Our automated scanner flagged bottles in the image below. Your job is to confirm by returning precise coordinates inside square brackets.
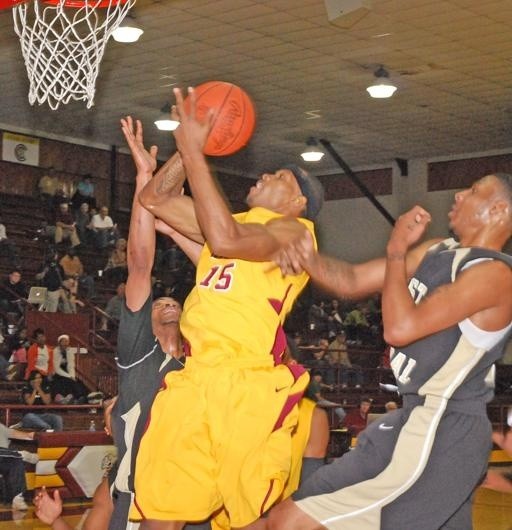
[89, 421, 96, 431]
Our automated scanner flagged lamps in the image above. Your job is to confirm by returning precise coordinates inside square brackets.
[108, 10, 145, 44]
[300, 137, 324, 162]
[366, 64, 397, 99]
[152, 102, 181, 131]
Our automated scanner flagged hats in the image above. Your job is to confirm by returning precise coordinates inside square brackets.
[281, 164, 325, 219]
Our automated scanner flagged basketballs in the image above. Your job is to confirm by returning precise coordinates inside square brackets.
[182, 83, 254, 156]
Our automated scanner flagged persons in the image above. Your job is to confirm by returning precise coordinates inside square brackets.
[1, 159, 511, 528]
[111, 115, 189, 528]
[129, 81, 326, 529]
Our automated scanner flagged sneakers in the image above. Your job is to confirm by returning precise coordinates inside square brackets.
[22, 451, 39, 464]
[12, 496, 28, 510]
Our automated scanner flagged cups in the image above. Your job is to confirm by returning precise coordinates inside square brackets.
[99, 270, 103, 276]
